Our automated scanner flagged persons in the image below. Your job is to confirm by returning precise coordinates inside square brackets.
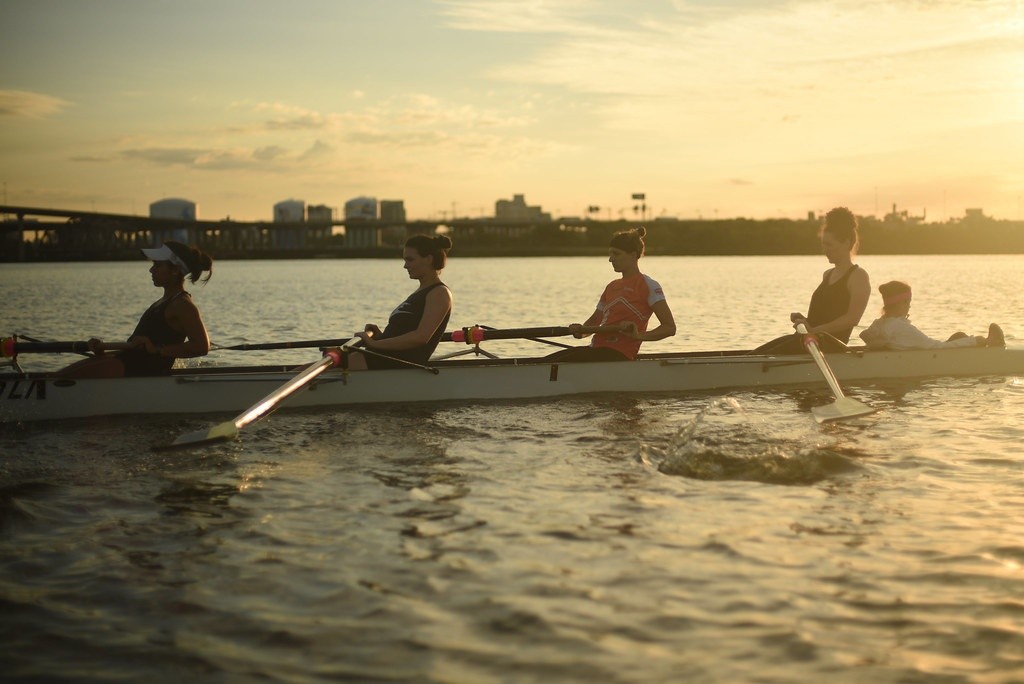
[289, 235, 453, 371]
[860, 281, 1005, 349]
[52, 241, 212, 377]
[538, 227, 676, 362]
[748, 208, 871, 355]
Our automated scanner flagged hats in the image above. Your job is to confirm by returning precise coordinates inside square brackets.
[140, 244, 191, 276]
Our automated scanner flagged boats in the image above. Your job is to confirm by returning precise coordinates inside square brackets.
[0, 345, 1024, 434]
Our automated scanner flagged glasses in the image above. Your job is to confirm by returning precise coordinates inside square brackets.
[152, 263, 160, 269]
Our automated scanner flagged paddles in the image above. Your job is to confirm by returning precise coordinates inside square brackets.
[794, 316, 876, 418]
[208, 322, 635, 353]
[148, 330, 375, 453]
[0, 337, 146, 357]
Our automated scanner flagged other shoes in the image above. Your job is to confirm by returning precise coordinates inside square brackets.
[986, 323, 1005, 346]
